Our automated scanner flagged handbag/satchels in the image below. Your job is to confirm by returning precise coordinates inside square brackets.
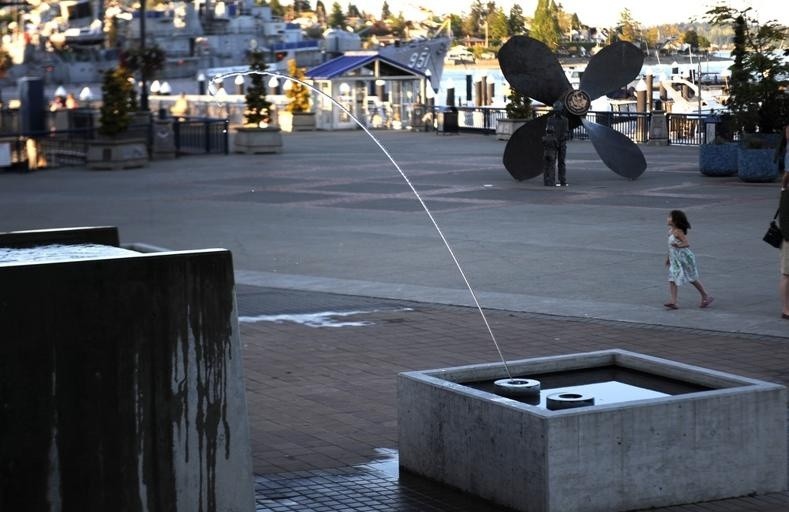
[763, 220, 782, 248]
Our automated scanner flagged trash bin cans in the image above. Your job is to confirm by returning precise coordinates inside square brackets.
[436, 105, 459, 137]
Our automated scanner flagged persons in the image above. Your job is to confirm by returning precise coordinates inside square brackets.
[546, 100, 569, 187]
[663, 209, 715, 310]
[542, 124, 560, 187]
[778, 170, 789, 319]
